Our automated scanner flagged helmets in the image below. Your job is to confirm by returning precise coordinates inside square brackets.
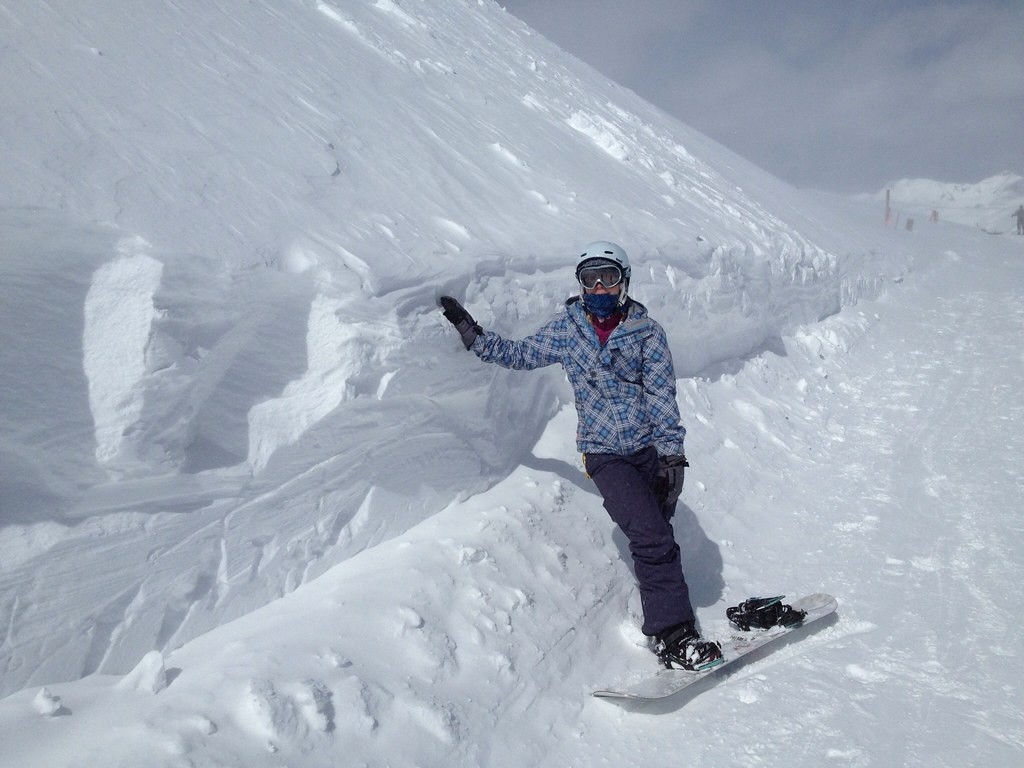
[575, 240, 631, 279]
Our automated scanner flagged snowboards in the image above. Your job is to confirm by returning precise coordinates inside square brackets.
[591, 589, 841, 705]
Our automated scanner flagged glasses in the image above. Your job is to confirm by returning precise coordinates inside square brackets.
[577, 265, 622, 289]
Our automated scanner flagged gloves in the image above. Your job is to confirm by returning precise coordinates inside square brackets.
[441, 295, 484, 351]
[653, 453, 690, 505]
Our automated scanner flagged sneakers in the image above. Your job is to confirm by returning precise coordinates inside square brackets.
[655, 622, 724, 671]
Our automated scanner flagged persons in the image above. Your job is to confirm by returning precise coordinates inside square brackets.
[1011, 205, 1024, 235]
[440, 239, 724, 671]
